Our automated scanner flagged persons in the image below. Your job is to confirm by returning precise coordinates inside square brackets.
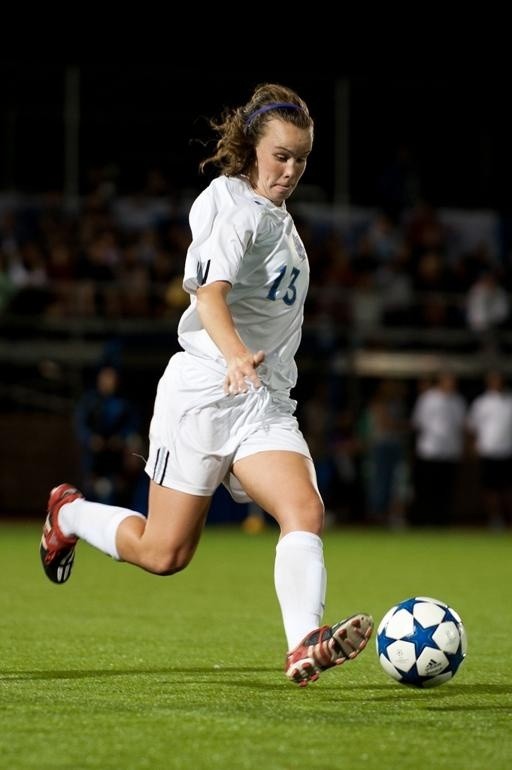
[37, 82, 374, 694]
[0, 174, 512, 530]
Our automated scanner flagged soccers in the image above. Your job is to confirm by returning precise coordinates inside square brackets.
[376, 596, 469, 689]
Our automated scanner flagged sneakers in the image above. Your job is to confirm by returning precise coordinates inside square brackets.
[283, 612, 375, 687]
[38, 483, 86, 584]
